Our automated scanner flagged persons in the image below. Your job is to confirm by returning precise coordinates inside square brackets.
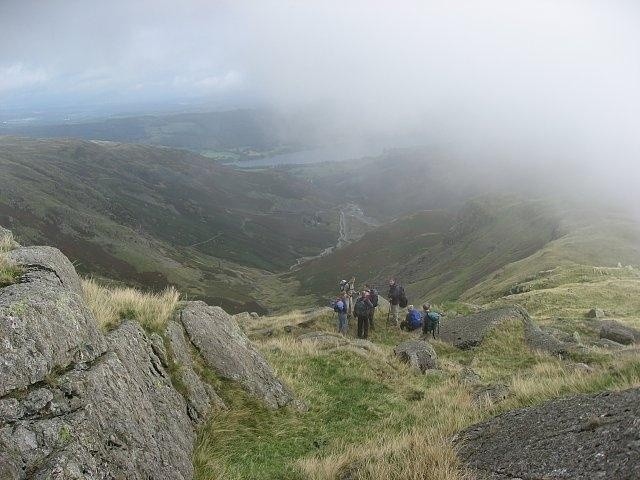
[335, 290, 353, 335]
[389, 278, 401, 327]
[342, 276, 360, 318]
[364, 284, 376, 331]
[418, 302, 433, 343]
[399, 305, 422, 332]
[353, 291, 374, 339]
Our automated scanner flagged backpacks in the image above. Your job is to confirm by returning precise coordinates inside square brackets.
[422, 312, 439, 333]
[353, 301, 370, 319]
[409, 310, 421, 328]
[371, 289, 378, 308]
[333, 296, 345, 312]
